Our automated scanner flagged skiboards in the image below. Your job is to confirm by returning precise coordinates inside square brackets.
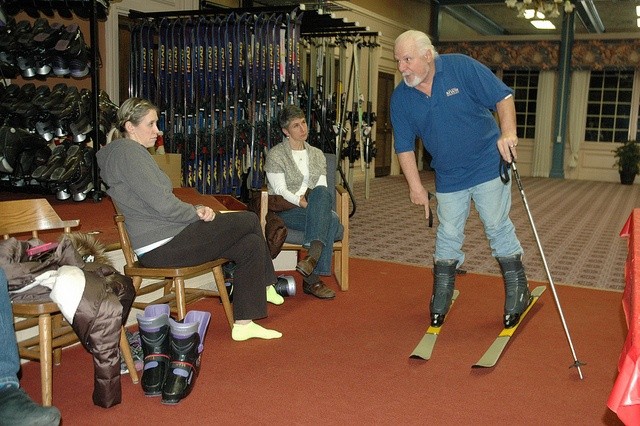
[409, 285, 547, 368]
[129, 12, 377, 203]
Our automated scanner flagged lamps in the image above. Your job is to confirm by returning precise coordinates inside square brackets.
[505, 0, 575, 21]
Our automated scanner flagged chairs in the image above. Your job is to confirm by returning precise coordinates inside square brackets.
[113, 214, 234, 332]
[257, 184, 350, 291]
[0, 198, 139, 407]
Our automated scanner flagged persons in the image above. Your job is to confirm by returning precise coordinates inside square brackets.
[96, 97, 285, 342]
[389, 29, 531, 316]
[0, 268, 62, 426]
[263, 104, 336, 299]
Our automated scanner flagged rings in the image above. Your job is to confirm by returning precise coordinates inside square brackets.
[514, 143, 517, 147]
[510, 145, 513, 148]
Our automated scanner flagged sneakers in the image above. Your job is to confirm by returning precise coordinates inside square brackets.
[0, 386, 61, 426]
[119, 332, 144, 375]
[0, 0, 117, 202]
[274, 275, 296, 297]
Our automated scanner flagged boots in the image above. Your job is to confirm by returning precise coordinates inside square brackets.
[495, 255, 531, 315]
[136, 304, 170, 396]
[162, 310, 211, 404]
[430, 259, 457, 316]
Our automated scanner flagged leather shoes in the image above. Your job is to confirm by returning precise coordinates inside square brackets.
[296, 255, 317, 277]
[303, 280, 336, 299]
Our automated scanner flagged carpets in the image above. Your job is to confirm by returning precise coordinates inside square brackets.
[1, 191, 627, 426]
